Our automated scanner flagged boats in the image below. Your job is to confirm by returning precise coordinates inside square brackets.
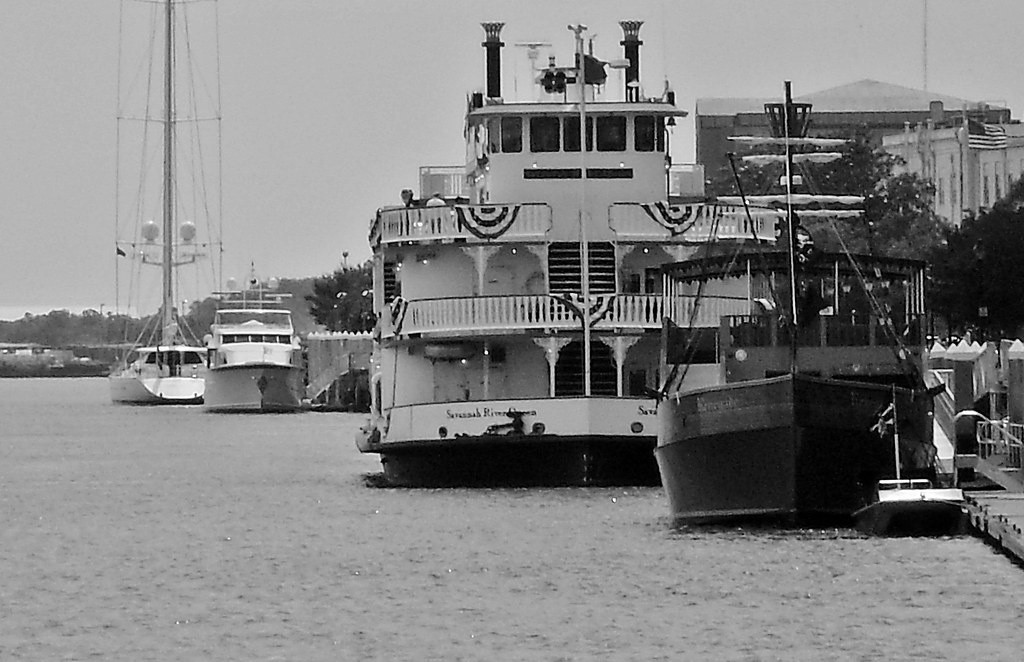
[49, 356, 111, 378]
[653, 101, 936, 533]
[354, 20, 777, 488]
[204, 261, 309, 413]
[850, 433, 964, 536]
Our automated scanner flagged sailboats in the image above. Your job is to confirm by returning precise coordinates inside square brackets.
[108, 1, 211, 403]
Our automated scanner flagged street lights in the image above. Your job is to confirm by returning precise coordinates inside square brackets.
[978, 303, 988, 344]
